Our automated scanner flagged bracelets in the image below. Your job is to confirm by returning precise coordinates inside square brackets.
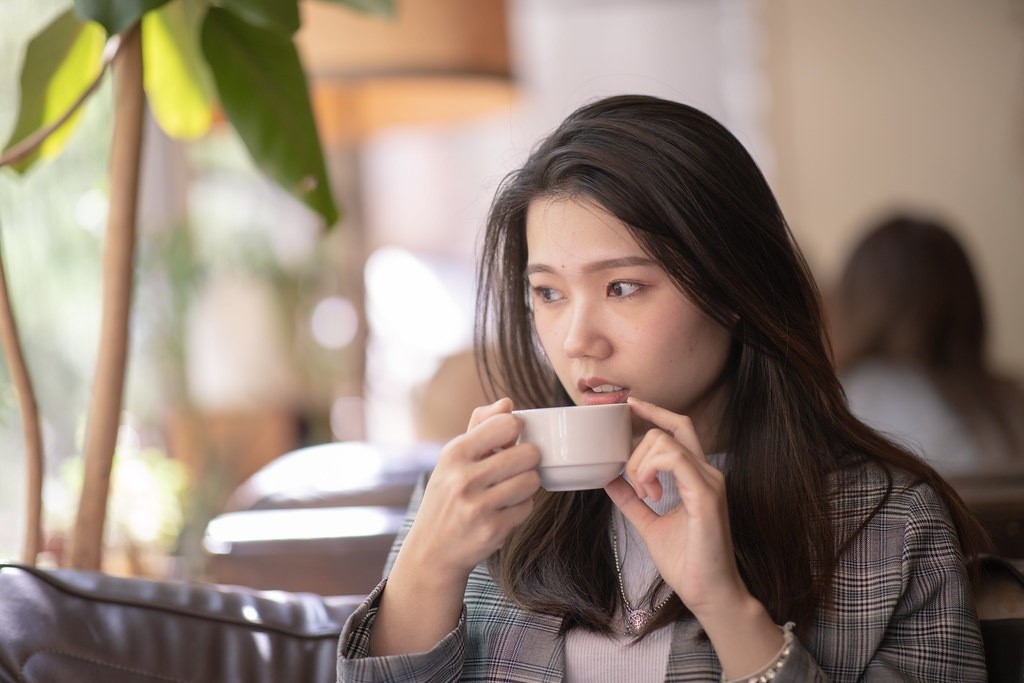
[741, 634, 790, 683]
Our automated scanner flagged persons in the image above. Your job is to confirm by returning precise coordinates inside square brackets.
[817, 205, 1024, 486]
[334, 93, 989, 683]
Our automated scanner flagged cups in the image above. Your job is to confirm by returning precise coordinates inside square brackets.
[503, 402, 634, 492]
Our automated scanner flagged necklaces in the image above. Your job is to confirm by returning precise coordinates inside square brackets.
[608, 502, 678, 636]
